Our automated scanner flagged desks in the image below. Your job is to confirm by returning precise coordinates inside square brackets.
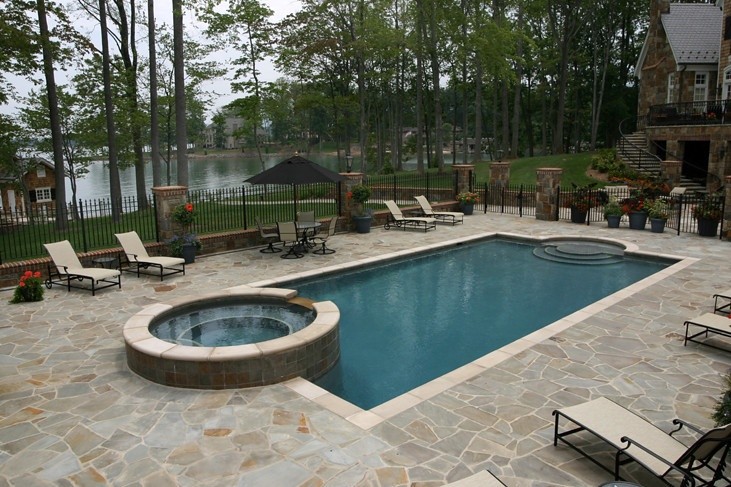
[276, 221, 321, 253]
[93, 257, 118, 280]
[598, 481, 644, 487]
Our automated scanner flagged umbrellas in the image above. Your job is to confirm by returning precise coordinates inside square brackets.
[243, 151, 347, 225]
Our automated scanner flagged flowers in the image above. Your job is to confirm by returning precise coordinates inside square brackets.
[346, 184, 372, 218]
[8, 271, 45, 304]
[571, 187, 721, 221]
[455, 190, 480, 205]
[170, 202, 203, 257]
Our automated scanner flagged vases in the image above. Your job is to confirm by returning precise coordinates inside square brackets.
[353, 217, 372, 233]
[461, 201, 474, 215]
[22, 287, 42, 300]
[571, 206, 718, 236]
[179, 244, 197, 264]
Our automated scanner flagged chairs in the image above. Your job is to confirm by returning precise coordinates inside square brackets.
[384, 195, 465, 233]
[552, 396, 731, 487]
[114, 230, 186, 281]
[440, 469, 508, 487]
[684, 288, 731, 353]
[255, 210, 338, 259]
[44, 239, 122, 296]
